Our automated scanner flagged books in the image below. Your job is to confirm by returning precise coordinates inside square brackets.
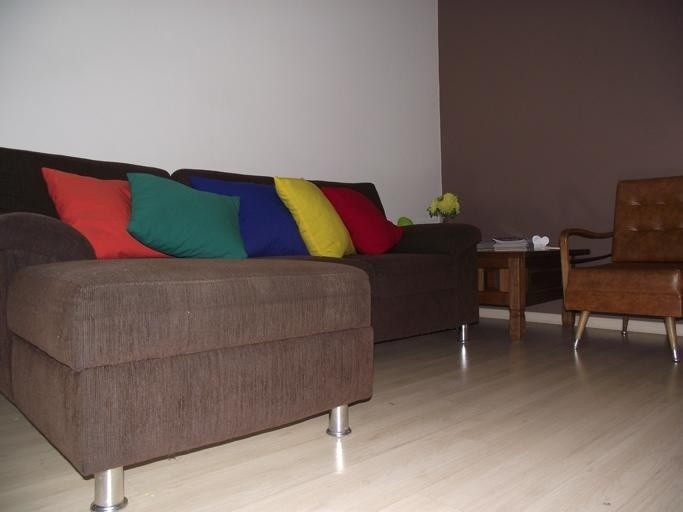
[476, 240, 495, 252]
[493, 236, 533, 250]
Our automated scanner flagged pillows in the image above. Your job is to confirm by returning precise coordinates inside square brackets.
[183, 172, 309, 260]
[121, 170, 251, 261]
[320, 184, 403, 256]
[38, 164, 176, 261]
[270, 173, 357, 259]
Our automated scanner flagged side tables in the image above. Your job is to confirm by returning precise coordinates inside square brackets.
[468, 245, 590, 343]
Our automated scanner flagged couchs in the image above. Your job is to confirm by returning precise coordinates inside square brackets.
[1, 146, 484, 404]
[0, 256, 374, 510]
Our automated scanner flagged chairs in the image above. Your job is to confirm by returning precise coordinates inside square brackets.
[556, 174, 683, 367]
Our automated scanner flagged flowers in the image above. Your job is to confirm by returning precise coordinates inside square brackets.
[422, 190, 462, 226]
